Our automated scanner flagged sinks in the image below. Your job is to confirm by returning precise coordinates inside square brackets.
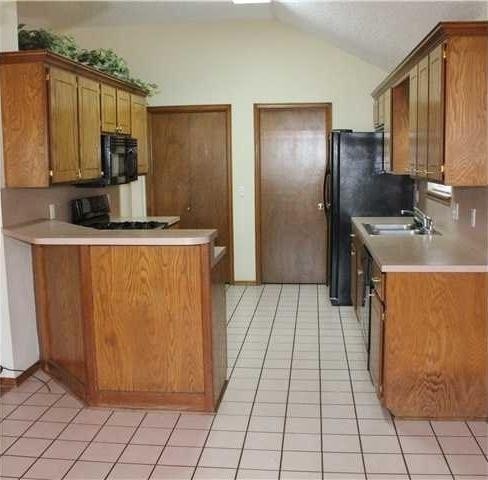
[367, 229, 417, 236]
[362, 223, 418, 229]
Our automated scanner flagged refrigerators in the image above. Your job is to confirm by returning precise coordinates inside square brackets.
[323, 130, 417, 307]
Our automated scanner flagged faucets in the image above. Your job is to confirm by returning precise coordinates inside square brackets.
[401, 209, 424, 224]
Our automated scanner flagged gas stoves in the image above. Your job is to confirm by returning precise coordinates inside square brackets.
[89, 219, 167, 230]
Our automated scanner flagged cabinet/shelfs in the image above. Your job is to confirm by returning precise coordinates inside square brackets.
[369, 21, 488, 186]
[101, 82, 131, 135]
[350, 223, 368, 323]
[369, 260, 385, 399]
[131, 92, 148, 175]
[0, 64, 101, 188]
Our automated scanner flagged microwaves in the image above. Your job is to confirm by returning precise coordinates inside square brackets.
[72, 133, 141, 188]
